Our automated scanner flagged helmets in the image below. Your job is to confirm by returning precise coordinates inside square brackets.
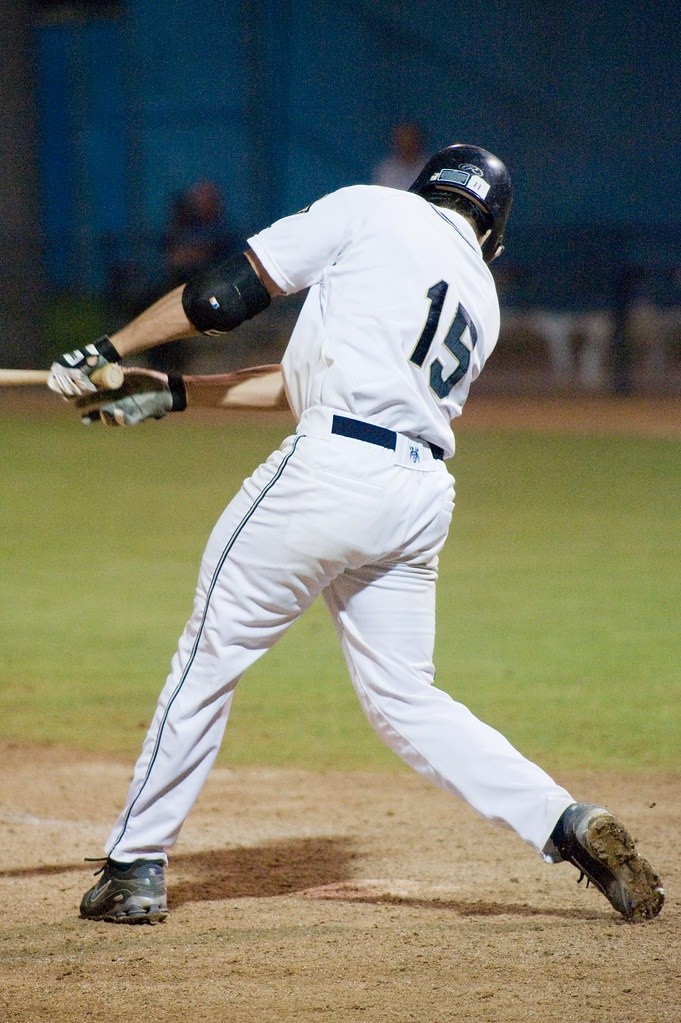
[407, 142, 513, 265]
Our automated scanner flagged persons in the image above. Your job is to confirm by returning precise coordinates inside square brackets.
[603, 228, 651, 397]
[375, 121, 430, 191]
[147, 187, 236, 373]
[48, 145, 666, 923]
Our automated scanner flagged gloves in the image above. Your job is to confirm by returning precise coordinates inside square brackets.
[47, 333, 124, 404]
[73, 363, 188, 430]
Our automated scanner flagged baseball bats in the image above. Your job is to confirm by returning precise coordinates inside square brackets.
[0, 362, 125, 392]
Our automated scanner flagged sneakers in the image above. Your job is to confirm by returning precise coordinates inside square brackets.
[548, 802, 666, 924]
[79, 854, 169, 925]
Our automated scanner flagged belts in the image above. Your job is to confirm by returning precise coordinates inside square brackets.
[331, 414, 448, 459]
